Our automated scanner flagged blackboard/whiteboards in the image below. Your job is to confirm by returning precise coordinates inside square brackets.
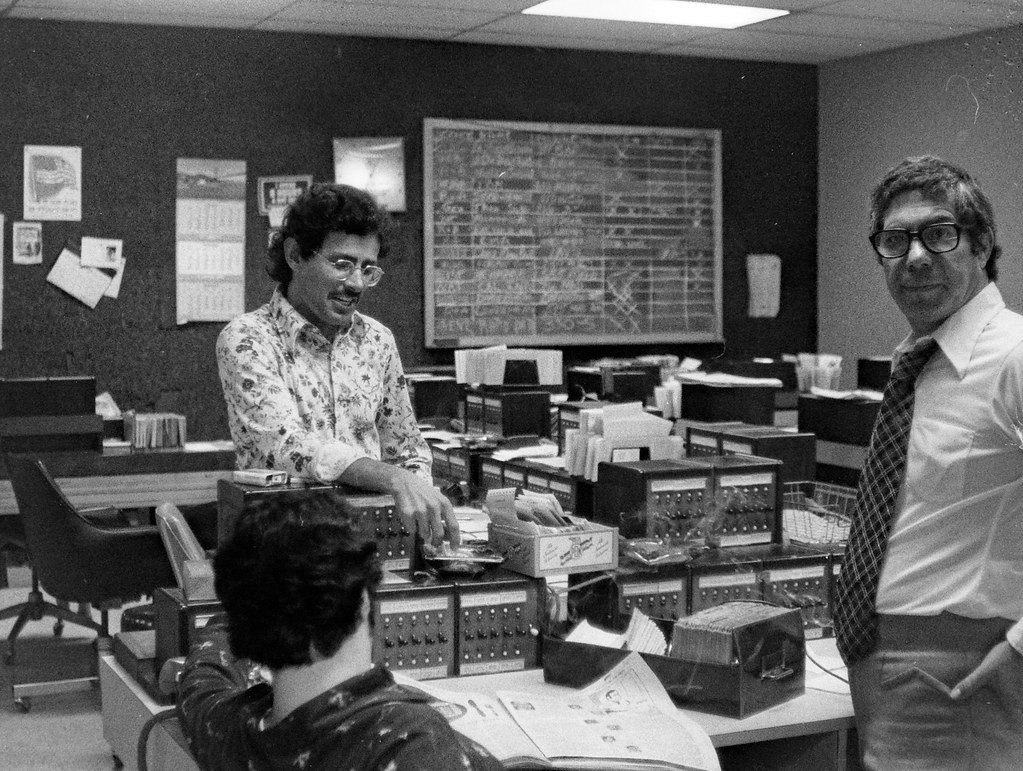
[422, 118, 726, 342]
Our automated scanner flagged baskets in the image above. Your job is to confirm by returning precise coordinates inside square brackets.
[783, 481, 857, 547]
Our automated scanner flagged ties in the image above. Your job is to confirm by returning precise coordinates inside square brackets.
[833, 339, 939, 666]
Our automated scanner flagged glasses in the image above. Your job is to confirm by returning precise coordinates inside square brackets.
[869, 222, 967, 259]
[313, 250, 385, 287]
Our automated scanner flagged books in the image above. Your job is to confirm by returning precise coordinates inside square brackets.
[390, 650, 721, 771]
[125, 410, 187, 447]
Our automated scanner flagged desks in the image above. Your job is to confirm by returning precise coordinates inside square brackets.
[0, 396, 893, 771]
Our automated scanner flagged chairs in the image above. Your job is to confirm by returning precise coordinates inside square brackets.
[11, 451, 177, 709]
[154, 503, 204, 603]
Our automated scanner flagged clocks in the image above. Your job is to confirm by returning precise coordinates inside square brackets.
[333, 137, 406, 212]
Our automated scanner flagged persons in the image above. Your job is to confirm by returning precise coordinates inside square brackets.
[176, 490, 509, 771]
[833, 155, 1023, 771]
[215, 184, 462, 552]
